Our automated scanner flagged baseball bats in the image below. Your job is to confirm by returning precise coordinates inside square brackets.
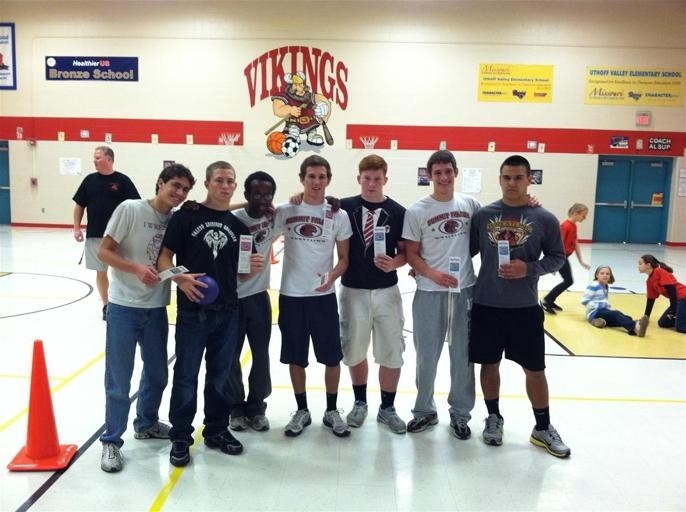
[264, 114, 293, 136]
[322, 120, 334, 146]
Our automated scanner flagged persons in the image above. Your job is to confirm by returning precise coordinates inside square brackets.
[272, 154, 354, 437]
[180, 171, 341, 432]
[638, 255, 686, 333]
[96, 163, 276, 473]
[580, 264, 649, 337]
[407, 155, 571, 458]
[290, 154, 407, 434]
[72, 146, 141, 321]
[400, 150, 542, 440]
[539, 203, 590, 317]
[157, 161, 264, 467]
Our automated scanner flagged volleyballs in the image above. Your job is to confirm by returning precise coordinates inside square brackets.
[311, 101, 329, 118]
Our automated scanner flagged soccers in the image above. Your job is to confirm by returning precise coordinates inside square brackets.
[281, 136, 300, 158]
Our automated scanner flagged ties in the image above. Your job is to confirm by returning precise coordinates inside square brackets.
[364, 211, 374, 246]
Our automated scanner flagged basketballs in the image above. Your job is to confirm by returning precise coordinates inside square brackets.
[267, 131, 286, 155]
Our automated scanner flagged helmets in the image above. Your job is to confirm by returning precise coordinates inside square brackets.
[283, 71, 305, 84]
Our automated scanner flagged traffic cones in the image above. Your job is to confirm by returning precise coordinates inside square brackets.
[8, 340, 77, 471]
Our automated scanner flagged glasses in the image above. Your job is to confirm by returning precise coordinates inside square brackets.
[252, 192, 273, 202]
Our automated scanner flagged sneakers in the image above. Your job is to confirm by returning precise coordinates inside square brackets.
[229, 416, 248, 431]
[101, 443, 122, 472]
[551, 302, 563, 312]
[407, 413, 438, 432]
[102, 305, 107, 321]
[529, 423, 571, 458]
[284, 409, 312, 436]
[134, 420, 171, 439]
[539, 300, 555, 314]
[449, 412, 472, 439]
[346, 401, 368, 428]
[482, 413, 504, 445]
[169, 441, 190, 467]
[249, 414, 270, 432]
[377, 404, 407, 434]
[203, 429, 243, 455]
[323, 407, 351, 436]
[592, 318, 606, 328]
[633, 315, 649, 337]
